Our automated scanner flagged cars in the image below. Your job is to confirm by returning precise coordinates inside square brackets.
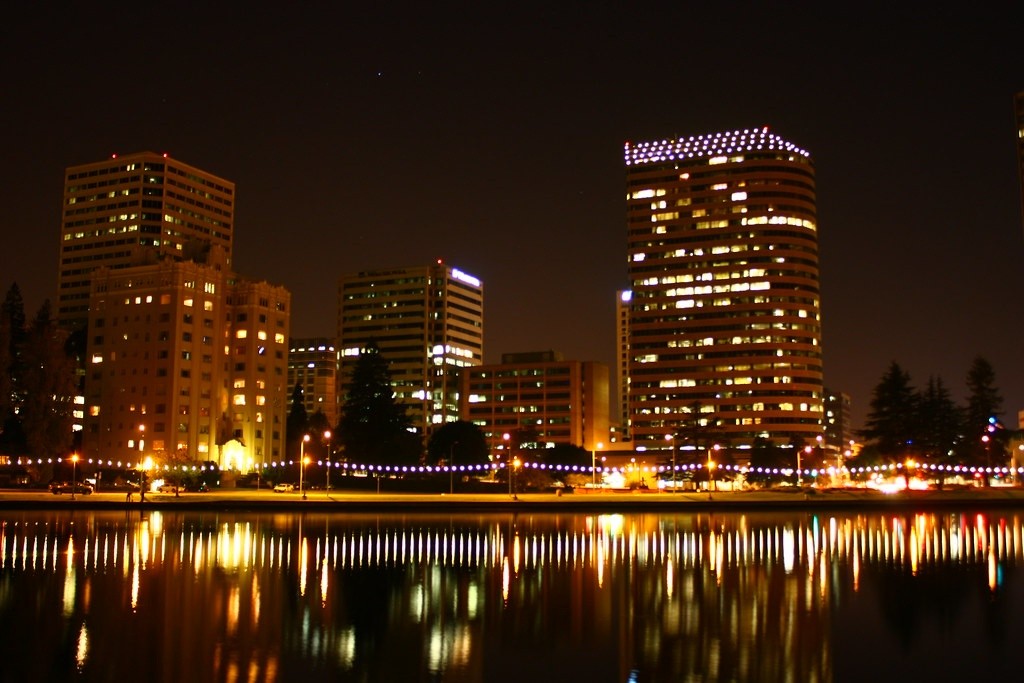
[49, 478, 95, 495]
[274, 482, 294, 494]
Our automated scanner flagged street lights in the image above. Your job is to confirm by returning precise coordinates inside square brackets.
[71, 455, 79, 498]
[299, 434, 311, 496]
[449, 440, 459, 495]
[140, 423, 145, 498]
[503, 432, 513, 496]
[665, 434, 676, 494]
[796, 445, 811, 485]
[592, 443, 603, 486]
[303, 456, 310, 500]
[707, 445, 721, 498]
[323, 431, 332, 495]
